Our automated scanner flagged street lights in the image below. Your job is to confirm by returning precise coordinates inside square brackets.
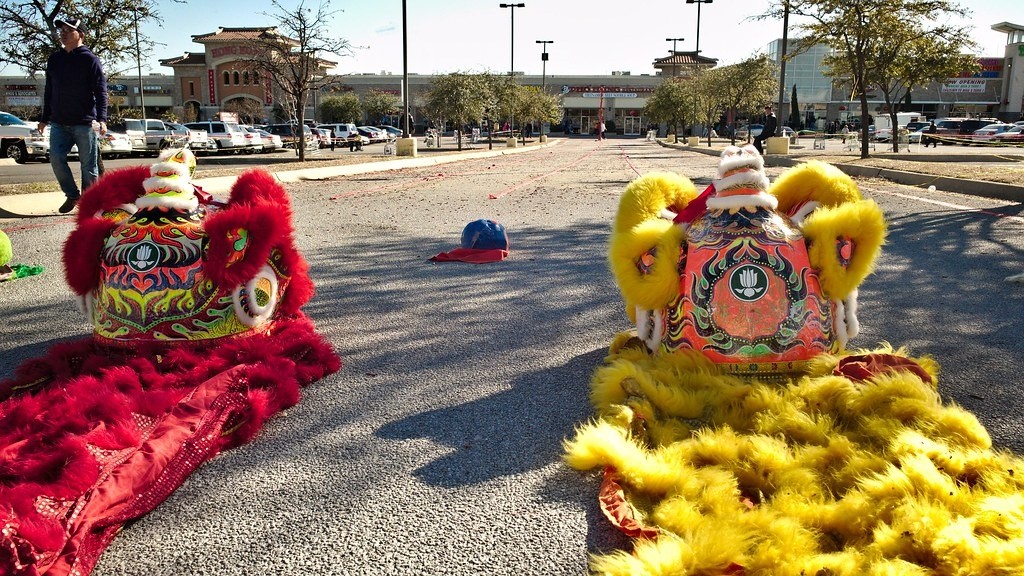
[686, 0, 716, 137]
[499, 3, 527, 138]
[665, 38, 685, 134]
[535, 39, 554, 136]
[305, 47, 320, 122]
[124, 5, 148, 120]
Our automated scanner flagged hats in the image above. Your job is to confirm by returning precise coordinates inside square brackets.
[55, 18, 85, 33]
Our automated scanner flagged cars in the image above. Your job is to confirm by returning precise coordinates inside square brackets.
[21, 117, 403, 164]
[858, 111, 1024, 146]
[734, 123, 764, 140]
[774, 126, 796, 137]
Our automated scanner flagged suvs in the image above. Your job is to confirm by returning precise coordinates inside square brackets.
[0, 110, 51, 165]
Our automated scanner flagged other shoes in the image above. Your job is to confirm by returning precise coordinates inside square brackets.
[59, 193, 81, 213]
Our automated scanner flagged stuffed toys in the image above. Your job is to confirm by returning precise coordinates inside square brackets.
[564, 145, 1024, 576]
[0, 146, 341, 576]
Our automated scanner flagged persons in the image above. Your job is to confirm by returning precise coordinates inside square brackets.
[37, 19, 108, 214]
[503, 122, 510, 131]
[924, 120, 936, 148]
[848, 123, 852, 132]
[330, 128, 336, 150]
[842, 125, 849, 143]
[527, 122, 532, 139]
[831, 122, 834, 138]
[399, 107, 415, 137]
[754, 106, 776, 155]
[591, 121, 607, 140]
[348, 132, 362, 152]
